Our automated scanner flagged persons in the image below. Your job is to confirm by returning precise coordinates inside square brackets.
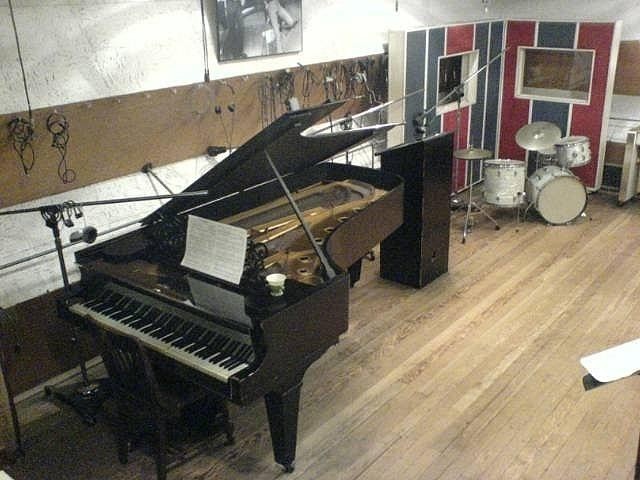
[264, 0, 295, 52]
[220, 0, 250, 56]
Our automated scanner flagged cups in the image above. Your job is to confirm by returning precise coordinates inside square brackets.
[264, 272, 286, 298]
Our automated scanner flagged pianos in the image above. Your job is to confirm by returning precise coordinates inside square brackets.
[56, 99, 406, 472]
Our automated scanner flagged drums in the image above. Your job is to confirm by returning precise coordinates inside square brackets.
[526, 165, 588, 225]
[554, 135, 592, 168]
[484, 159, 527, 209]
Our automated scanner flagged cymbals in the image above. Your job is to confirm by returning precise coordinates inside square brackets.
[515, 121, 562, 151]
[453, 148, 494, 160]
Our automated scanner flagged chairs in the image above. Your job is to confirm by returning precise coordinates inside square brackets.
[86, 324, 236, 480]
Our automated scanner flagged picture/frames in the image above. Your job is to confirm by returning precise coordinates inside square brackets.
[216, 0, 303, 62]
